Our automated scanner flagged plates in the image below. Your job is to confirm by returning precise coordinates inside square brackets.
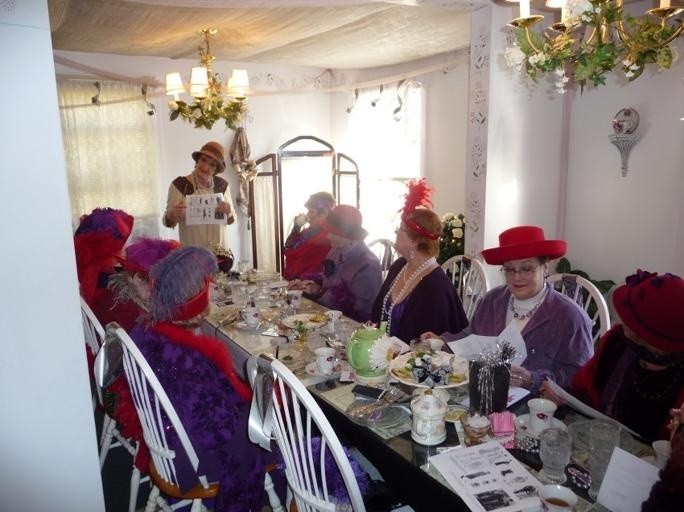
[347, 399, 407, 426]
[443, 404, 470, 423]
[568, 421, 634, 455]
[514, 415, 567, 439]
[387, 350, 470, 388]
[305, 360, 344, 375]
[282, 311, 326, 330]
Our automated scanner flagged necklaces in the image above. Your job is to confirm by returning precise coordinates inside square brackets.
[510, 290, 546, 320]
[381, 256, 436, 335]
[634, 364, 683, 399]
[192, 170, 214, 194]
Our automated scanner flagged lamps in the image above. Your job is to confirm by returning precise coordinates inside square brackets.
[500, 0, 684, 100]
[158, 29, 251, 132]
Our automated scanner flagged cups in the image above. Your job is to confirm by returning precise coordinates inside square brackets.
[527, 398, 556, 431]
[588, 418, 621, 501]
[409, 337, 444, 352]
[213, 269, 302, 337]
[314, 347, 335, 372]
[324, 310, 351, 344]
[537, 428, 571, 482]
[460, 410, 492, 445]
[537, 484, 577, 512]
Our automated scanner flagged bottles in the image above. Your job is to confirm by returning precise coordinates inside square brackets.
[409, 388, 447, 445]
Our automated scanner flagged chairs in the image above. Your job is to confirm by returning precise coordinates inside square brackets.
[76, 236, 684, 512]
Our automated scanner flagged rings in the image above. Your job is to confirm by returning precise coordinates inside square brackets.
[540, 389, 545, 395]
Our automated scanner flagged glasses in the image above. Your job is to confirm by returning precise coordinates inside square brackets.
[497, 263, 542, 276]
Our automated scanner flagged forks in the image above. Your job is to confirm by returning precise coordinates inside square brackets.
[349, 387, 407, 417]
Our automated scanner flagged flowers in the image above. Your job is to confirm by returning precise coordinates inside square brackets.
[440, 211, 464, 256]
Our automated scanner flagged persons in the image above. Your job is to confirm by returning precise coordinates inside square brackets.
[420, 225, 594, 398]
[539, 268, 684, 448]
[287, 205, 382, 322]
[371, 208, 469, 345]
[91, 237, 184, 478]
[641, 402, 684, 512]
[162, 141, 237, 254]
[129, 244, 370, 512]
[282, 191, 336, 281]
[73, 207, 134, 326]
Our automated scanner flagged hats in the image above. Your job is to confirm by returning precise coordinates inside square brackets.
[191, 141, 226, 173]
[316, 205, 368, 241]
[604, 268, 684, 354]
[481, 226, 567, 265]
[111, 236, 182, 277]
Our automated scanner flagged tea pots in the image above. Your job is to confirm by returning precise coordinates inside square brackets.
[346, 320, 394, 379]
